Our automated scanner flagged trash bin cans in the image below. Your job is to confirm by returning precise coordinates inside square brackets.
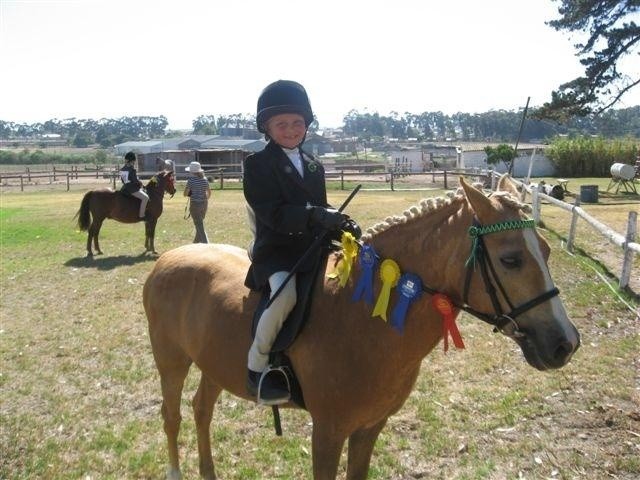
[581, 185, 598, 203]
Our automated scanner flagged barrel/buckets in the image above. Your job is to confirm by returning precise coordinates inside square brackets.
[580, 184, 599, 204]
[610, 162, 636, 180]
[530, 182, 565, 201]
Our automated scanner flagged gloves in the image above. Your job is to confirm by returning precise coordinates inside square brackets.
[330, 211, 363, 243]
[311, 204, 344, 233]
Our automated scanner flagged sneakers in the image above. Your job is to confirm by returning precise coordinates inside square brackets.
[245, 368, 288, 399]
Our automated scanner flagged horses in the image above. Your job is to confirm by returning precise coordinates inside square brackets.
[142, 171, 583, 479]
[71, 171, 177, 258]
[155, 156, 173, 171]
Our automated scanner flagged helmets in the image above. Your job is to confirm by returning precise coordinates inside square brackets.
[125, 151, 137, 162]
[253, 78, 316, 134]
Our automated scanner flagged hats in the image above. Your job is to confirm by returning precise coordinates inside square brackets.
[184, 161, 205, 174]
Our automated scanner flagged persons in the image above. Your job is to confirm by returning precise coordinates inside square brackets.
[119, 152, 150, 220]
[242, 80, 350, 400]
[184, 162, 212, 243]
